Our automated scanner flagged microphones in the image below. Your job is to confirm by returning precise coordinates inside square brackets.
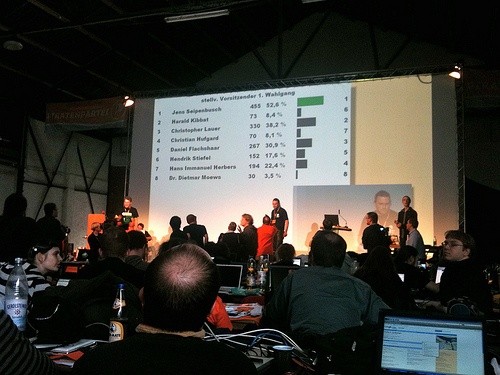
[339, 209, 348, 228]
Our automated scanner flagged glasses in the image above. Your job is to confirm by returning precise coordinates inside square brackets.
[441, 242, 463, 247]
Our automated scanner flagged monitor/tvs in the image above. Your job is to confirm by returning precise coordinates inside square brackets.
[433, 265, 446, 284]
[268, 265, 303, 292]
[215, 264, 243, 292]
[375, 308, 489, 375]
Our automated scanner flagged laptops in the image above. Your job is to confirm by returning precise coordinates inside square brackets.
[323, 215, 348, 227]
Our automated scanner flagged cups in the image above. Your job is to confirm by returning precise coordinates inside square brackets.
[274, 346, 292, 373]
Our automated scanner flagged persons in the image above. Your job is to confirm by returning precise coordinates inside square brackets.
[259, 196, 491, 352]
[357, 190, 401, 248]
[0, 193, 257, 375]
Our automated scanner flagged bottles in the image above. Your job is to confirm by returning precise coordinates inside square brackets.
[4, 257, 29, 330]
[434, 236, 437, 245]
[109, 284, 130, 341]
[246, 263, 256, 288]
[257, 256, 266, 288]
